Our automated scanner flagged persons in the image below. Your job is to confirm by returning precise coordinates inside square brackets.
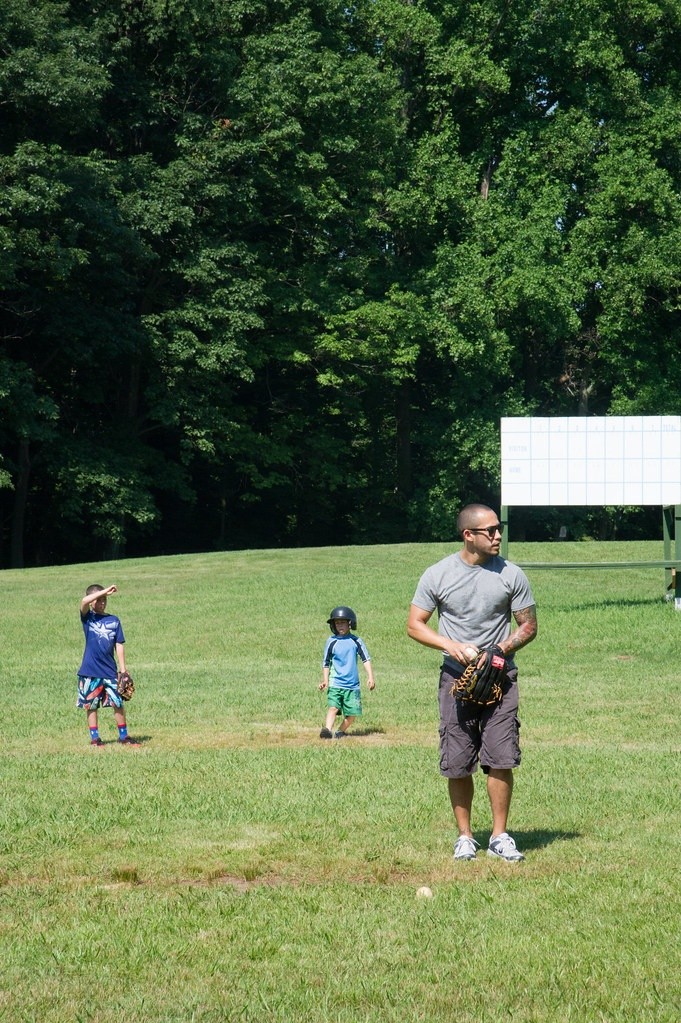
[319, 606, 375, 739]
[407, 504, 537, 864]
[76, 584, 141, 748]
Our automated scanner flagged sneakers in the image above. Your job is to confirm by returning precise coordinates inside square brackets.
[453, 835, 481, 862]
[91, 737, 106, 748]
[486, 832, 528, 863]
[117, 735, 142, 748]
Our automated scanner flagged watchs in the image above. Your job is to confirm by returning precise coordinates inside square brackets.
[493, 644, 504, 657]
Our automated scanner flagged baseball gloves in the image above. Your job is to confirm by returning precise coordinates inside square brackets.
[448, 642, 508, 710]
[116, 670, 136, 702]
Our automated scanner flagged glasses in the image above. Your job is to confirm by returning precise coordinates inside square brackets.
[461, 523, 505, 536]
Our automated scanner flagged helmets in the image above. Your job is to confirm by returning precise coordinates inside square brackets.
[326, 606, 358, 636]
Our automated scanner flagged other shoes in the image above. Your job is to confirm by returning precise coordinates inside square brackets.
[334, 730, 347, 738]
[320, 727, 332, 738]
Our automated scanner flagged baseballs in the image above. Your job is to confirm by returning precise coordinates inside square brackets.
[465, 647, 477, 664]
